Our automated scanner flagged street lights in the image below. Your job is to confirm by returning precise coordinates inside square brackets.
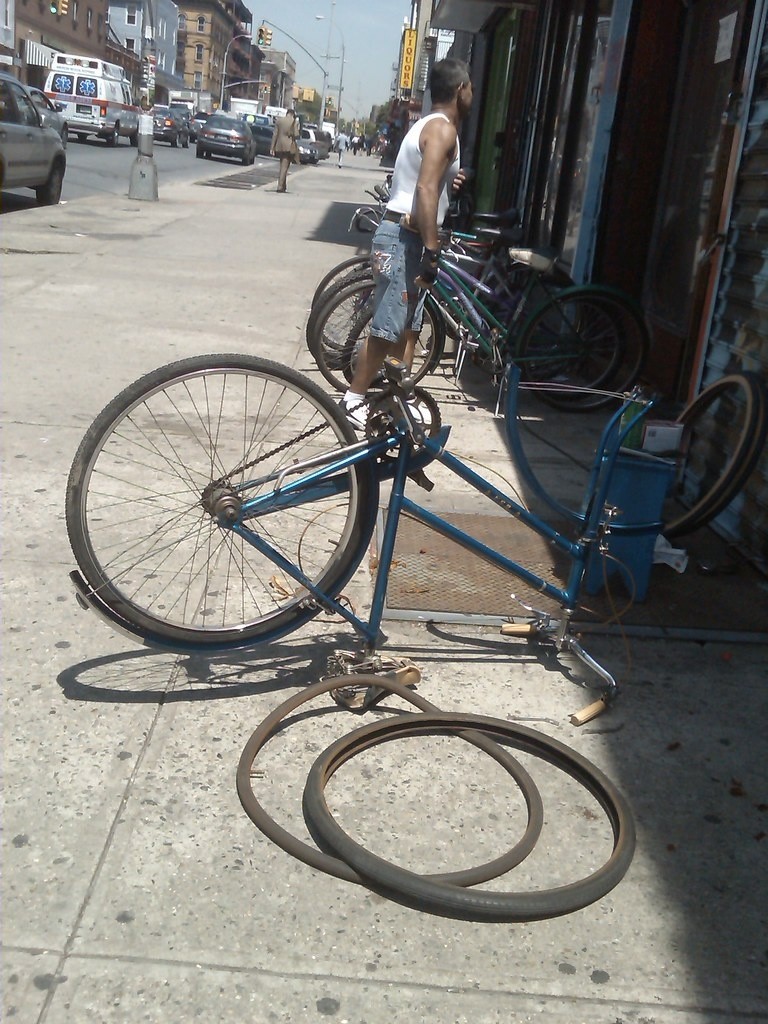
[219, 34, 253, 110]
[315, 15, 345, 138]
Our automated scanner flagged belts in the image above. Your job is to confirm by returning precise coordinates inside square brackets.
[383, 211, 401, 222]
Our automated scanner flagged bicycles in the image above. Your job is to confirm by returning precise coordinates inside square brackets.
[62, 352, 667, 726]
[306, 173, 651, 415]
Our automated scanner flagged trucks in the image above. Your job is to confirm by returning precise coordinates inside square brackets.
[230, 97, 262, 118]
[168, 90, 212, 115]
[263, 105, 287, 118]
[322, 121, 336, 146]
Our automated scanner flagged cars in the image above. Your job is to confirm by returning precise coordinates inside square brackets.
[196, 113, 256, 166]
[295, 122, 333, 165]
[22, 85, 70, 151]
[252, 125, 274, 155]
[1, 73, 67, 206]
[148, 105, 211, 148]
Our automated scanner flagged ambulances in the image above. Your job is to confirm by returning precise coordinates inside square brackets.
[44, 54, 139, 148]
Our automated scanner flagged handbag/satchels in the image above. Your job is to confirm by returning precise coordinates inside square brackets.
[290, 141, 301, 165]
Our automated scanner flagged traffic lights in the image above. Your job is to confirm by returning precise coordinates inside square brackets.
[57, 0, 68, 15]
[264, 85, 271, 94]
[263, 28, 273, 47]
[257, 26, 266, 45]
[50, 0, 58, 14]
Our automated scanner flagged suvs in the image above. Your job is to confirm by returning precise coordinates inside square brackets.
[242, 113, 270, 127]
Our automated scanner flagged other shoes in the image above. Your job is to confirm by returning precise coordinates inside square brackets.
[337, 392, 379, 432]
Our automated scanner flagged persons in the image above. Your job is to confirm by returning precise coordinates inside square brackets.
[336, 57, 473, 428]
[348, 134, 363, 155]
[270, 109, 299, 192]
[378, 135, 388, 163]
[336, 130, 349, 168]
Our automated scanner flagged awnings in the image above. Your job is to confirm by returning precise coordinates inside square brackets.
[24, 38, 62, 67]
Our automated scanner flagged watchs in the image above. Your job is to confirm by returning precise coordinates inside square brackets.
[424, 247, 441, 260]
